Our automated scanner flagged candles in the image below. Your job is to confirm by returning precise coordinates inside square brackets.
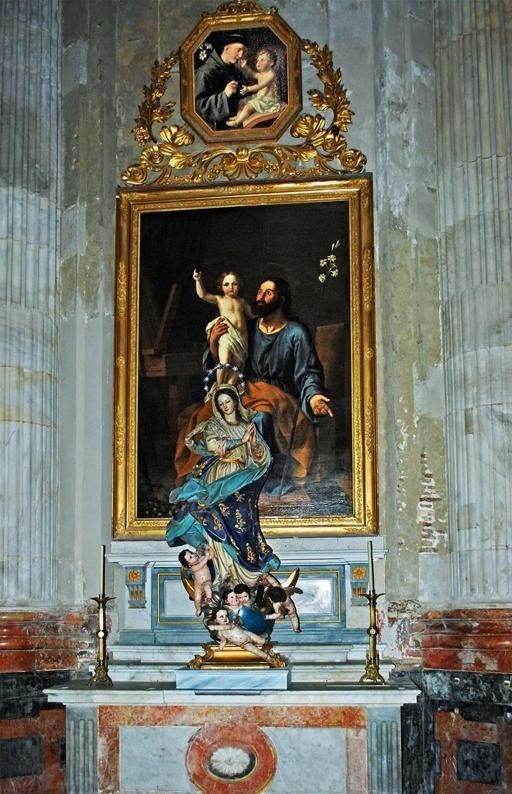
[100, 544, 105, 592]
[368, 541, 375, 590]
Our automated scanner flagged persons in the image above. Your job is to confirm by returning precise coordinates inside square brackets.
[191, 266, 262, 385]
[224, 46, 285, 127]
[207, 569, 304, 663]
[193, 33, 250, 129]
[166, 382, 280, 581]
[179, 542, 216, 617]
[200, 274, 334, 496]
